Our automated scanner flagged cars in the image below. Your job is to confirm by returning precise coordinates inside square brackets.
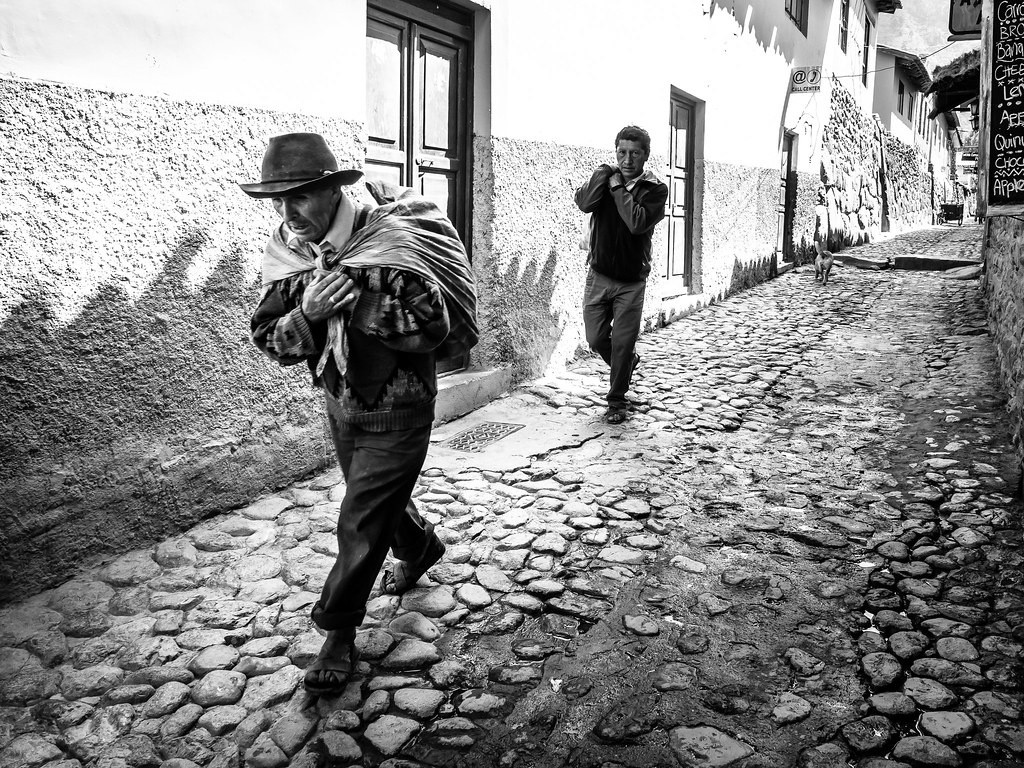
[962, 154, 979, 174]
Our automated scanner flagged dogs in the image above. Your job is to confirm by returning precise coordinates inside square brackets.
[814, 241, 833, 285]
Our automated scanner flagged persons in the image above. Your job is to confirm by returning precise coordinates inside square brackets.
[238, 132, 450, 695]
[574, 126, 668, 424]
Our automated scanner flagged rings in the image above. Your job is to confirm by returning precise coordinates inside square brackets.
[329, 296, 335, 303]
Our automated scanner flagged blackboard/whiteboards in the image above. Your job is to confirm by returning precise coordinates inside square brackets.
[975, 0, 1024, 216]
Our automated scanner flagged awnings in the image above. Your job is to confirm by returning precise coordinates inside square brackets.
[924, 51, 980, 120]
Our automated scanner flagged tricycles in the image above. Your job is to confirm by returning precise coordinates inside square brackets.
[936, 203, 965, 226]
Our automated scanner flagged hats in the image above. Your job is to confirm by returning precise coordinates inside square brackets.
[239, 133, 363, 198]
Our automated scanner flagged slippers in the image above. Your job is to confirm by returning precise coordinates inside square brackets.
[380, 546, 446, 596]
[626, 353, 639, 391]
[303, 633, 361, 695]
[606, 403, 626, 424]
